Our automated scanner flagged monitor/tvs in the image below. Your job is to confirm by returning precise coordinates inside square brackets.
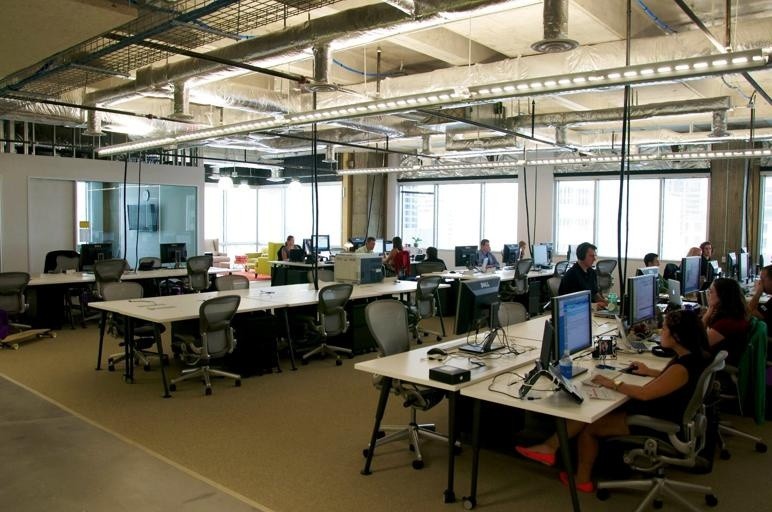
[126, 204, 156, 232]
[160, 243, 187, 269]
[310, 233, 394, 253]
[75, 241, 113, 273]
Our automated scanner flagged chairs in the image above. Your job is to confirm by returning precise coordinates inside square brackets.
[663, 263, 680, 280]
[203, 238, 287, 278]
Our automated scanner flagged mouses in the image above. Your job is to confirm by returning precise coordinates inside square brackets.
[428, 348, 449, 356]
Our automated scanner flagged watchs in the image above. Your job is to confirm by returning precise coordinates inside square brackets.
[613, 380, 624, 391]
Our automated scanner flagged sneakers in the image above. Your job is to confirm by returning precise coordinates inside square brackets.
[560, 472, 594, 493]
[515, 446, 556, 466]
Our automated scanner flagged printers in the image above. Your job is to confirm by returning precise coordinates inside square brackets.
[334, 252, 384, 285]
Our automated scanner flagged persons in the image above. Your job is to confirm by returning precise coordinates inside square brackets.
[678, 247, 704, 269]
[281, 235, 300, 268]
[472, 238, 501, 270]
[746, 266, 771, 362]
[643, 253, 661, 267]
[354, 236, 377, 254]
[699, 240, 714, 277]
[558, 242, 609, 311]
[514, 309, 711, 494]
[380, 236, 404, 277]
[516, 241, 527, 261]
[700, 275, 752, 396]
[422, 246, 448, 271]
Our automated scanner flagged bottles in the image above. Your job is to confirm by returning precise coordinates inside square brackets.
[606, 289, 618, 313]
[685, 304, 693, 310]
[558, 349, 574, 378]
